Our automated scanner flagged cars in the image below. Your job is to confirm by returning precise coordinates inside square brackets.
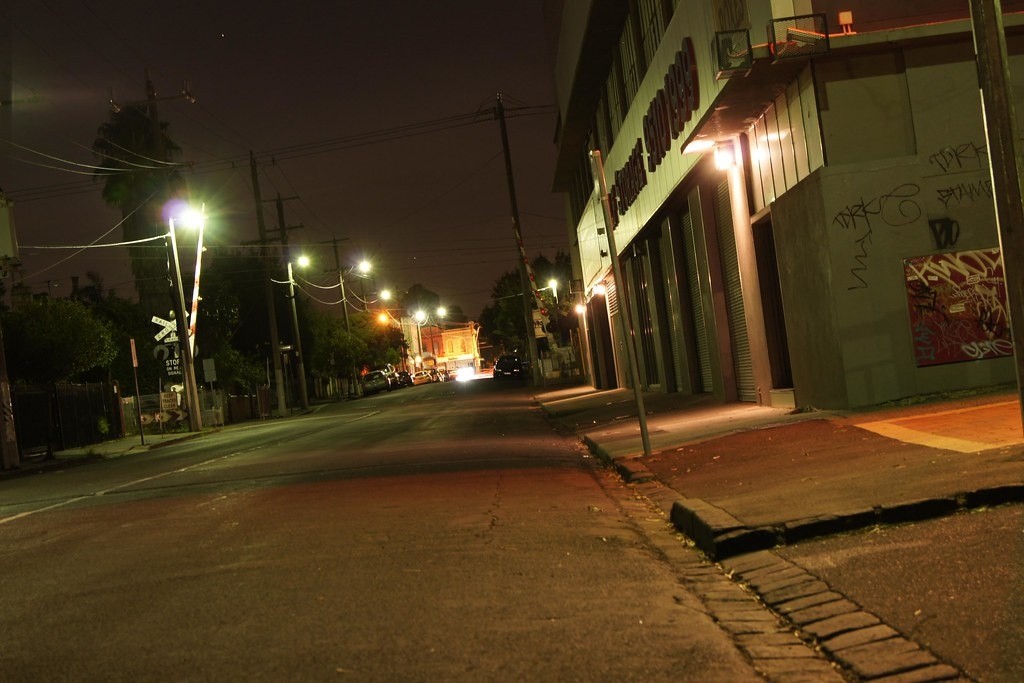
[359, 362, 459, 396]
[492, 352, 525, 379]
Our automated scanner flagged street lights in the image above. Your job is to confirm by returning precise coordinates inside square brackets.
[548, 278, 567, 377]
[412, 309, 427, 372]
[359, 288, 392, 311]
[426, 307, 448, 364]
[337, 260, 373, 396]
[167, 207, 203, 430]
[286, 253, 312, 410]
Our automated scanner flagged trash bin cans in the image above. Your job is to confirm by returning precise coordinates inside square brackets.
[204, 406, 224, 427]
